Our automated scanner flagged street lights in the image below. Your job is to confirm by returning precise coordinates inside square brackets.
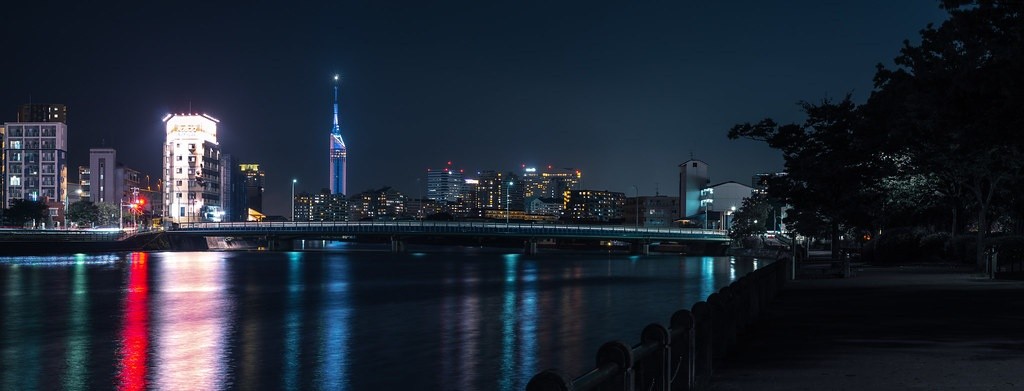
[631, 185, 639, 227]
[507, 182, 513, 223]
[291, 179, 297, 221]
[66, 188, 83, 228]
[417, 177, 422, 221]
[119, 198, 133, 229]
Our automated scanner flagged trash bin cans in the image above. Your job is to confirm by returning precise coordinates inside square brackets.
[984, 247, 999, 280]
